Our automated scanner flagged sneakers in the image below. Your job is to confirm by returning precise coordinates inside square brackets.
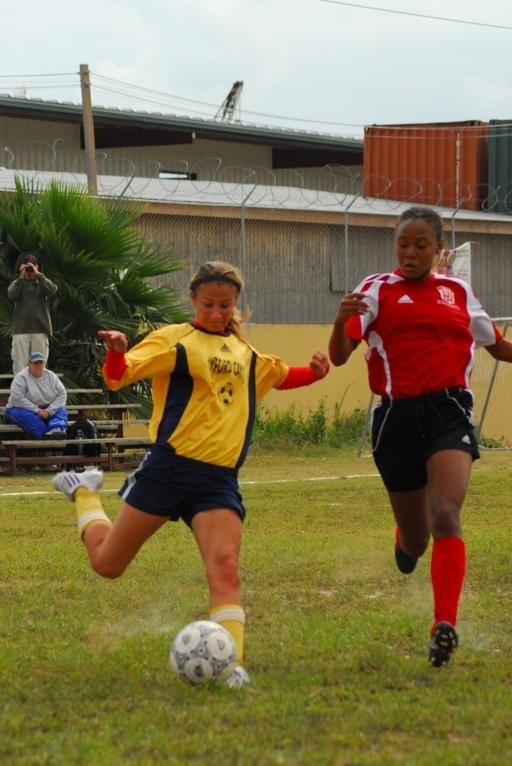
[429, 622, 458, 667]
[52, 466, 103, 502]
[46, 429, 65, 439]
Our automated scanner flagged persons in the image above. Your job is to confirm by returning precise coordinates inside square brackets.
[329, 207, 511, 664]
[8, 254, 60, 379]
[5, 352, 69, 440]
[52, 260, 331, 690]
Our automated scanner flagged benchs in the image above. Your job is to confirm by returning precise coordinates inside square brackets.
[0, 372, 154, 474]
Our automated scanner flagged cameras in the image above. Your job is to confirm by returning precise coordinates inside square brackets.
[25, 265, 34, 272]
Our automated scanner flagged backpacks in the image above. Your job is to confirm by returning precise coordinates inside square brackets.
[65, 417, 101, 456]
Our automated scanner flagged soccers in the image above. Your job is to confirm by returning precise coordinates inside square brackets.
[170, 621, 236, 690]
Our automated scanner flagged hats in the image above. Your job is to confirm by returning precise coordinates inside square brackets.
[30, 352, 44, 363]
